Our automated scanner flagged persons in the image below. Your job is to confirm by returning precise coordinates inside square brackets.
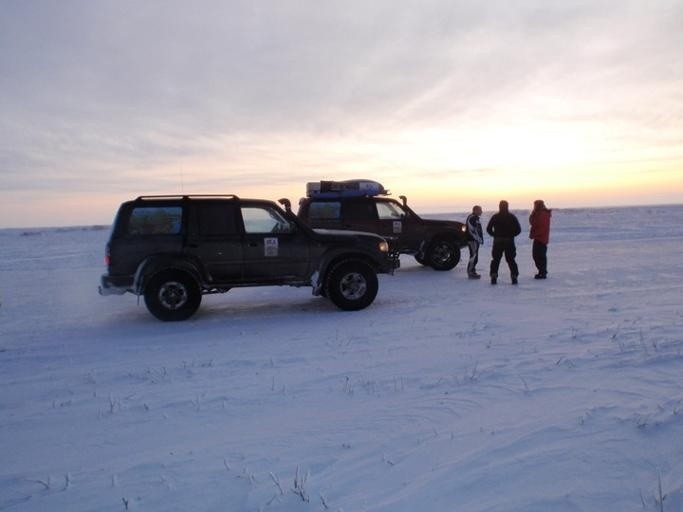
[487, 200, 521, 285]
[529, 200, 552, 279]
[465, 205, 484, 279]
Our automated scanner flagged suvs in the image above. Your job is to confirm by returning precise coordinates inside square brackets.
[99, 196, 400, 322]
[298, 196, 467, 270]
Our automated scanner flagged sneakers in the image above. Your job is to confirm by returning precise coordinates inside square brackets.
[535, 273, 546, 278]
[468, 274, 480, 279]
